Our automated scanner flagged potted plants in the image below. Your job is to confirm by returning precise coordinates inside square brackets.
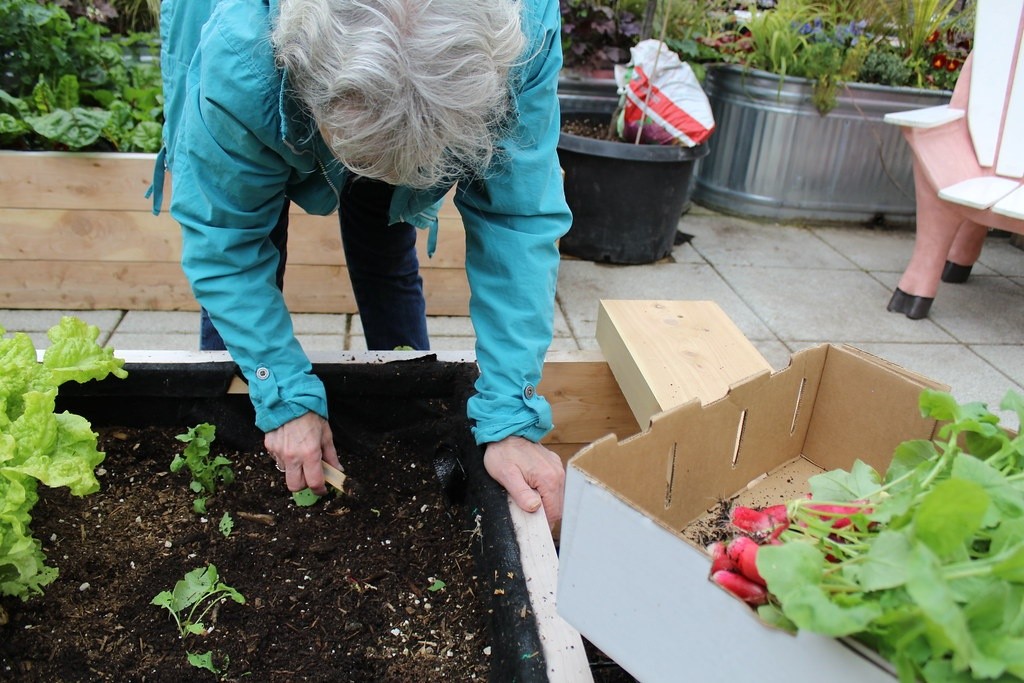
[0, 1, 472, 315]
[0, 316, 594, 683]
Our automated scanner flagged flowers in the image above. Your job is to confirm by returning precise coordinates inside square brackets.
[713, 1, 976, 118]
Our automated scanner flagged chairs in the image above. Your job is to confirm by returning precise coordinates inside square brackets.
[888, 1, 1024, 319]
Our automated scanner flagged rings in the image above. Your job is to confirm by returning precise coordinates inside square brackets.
[275, 461, 285, 473]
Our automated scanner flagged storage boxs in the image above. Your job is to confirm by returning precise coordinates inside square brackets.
[558, 343, 1018, 683]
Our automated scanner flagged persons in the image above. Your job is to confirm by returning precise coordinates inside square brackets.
[159, 0, 574, 548]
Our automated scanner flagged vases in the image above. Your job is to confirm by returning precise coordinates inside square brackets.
[690, 62, 953, 227]
[557, 95, 710, 266]
